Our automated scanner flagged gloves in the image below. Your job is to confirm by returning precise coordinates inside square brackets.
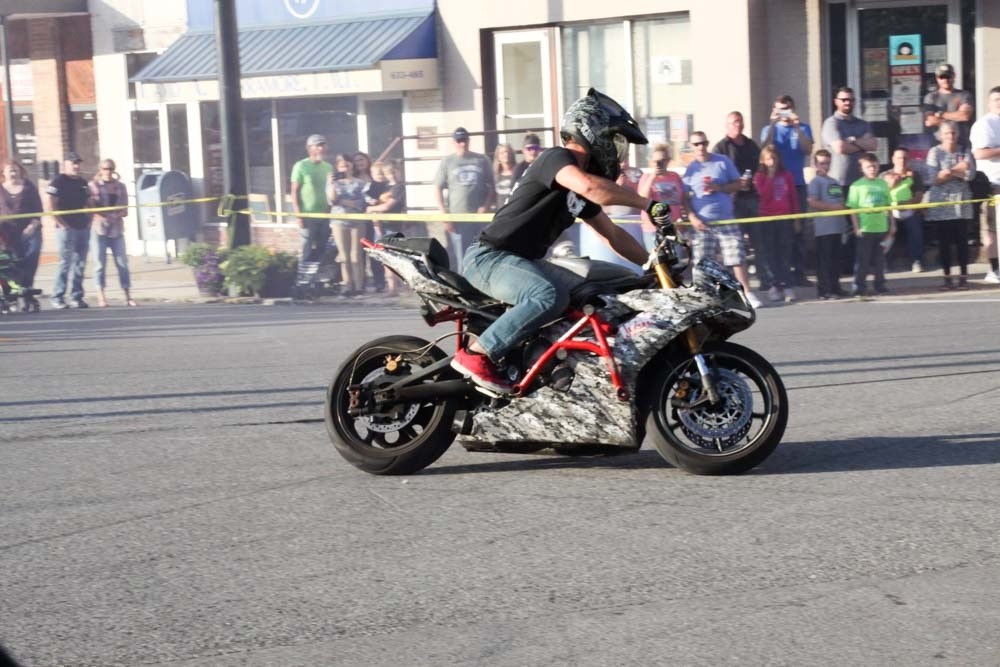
[646, 200, 672, 228]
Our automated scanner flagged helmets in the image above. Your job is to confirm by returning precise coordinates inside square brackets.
[559, 87, 650, 182]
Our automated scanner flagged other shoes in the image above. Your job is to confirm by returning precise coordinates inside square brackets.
[49, 297, 68, 309]
[68, 298, 88, 308]
[349, 291, 363, 300]
[736, 269, 1000, 307]
[337, 289, 352, 299]
[127, 299, 138, 307]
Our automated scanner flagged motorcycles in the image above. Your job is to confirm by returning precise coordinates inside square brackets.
[324, 200, 789, 476]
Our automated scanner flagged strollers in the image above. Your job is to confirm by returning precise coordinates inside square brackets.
[0, 250, 42, 314]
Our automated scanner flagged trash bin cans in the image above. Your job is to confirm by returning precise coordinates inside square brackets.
[134, 170, 191, 242]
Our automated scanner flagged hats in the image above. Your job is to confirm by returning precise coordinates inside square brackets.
[306, 135, 328, 146]
[522, 133, 541, 146]
[934, 64, 954, 76]
[452, 126, 470, 140]
[64, 152, 84, 164]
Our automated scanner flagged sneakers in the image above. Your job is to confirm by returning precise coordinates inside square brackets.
[450, 347, 514, 394]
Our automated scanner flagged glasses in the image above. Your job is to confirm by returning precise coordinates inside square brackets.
[455, 138, 468, 143]
[836, 97, 853, 103]
[692, 140, 710, 147]
[938, 75, 951, 80]
[525, 146, 540, 151]
[101, 167, 115, 171]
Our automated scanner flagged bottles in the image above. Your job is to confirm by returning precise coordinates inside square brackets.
[740, 169, 753, 191]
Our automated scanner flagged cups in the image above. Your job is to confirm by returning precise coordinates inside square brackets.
[700, 176, 712, 196]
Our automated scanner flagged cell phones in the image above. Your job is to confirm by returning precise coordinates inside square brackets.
[332, 172, 346, 180]
[778, 109, 792, 118]
[656, 160, 664, 169]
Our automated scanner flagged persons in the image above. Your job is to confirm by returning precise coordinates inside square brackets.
[292, 133, 405, 293]
[709, 86, 1000, 303]
[922, 64, 974, 150]
[46, 151, 94, 308]
[450, 87, 674, 394]
[87, 158, 138, 308]
[0, 162, 43, 312]
[437, 128, 544, 276]
[613, 130, 762, 308]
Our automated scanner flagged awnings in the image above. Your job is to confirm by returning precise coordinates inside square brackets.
[123, 14, 442, 108]
